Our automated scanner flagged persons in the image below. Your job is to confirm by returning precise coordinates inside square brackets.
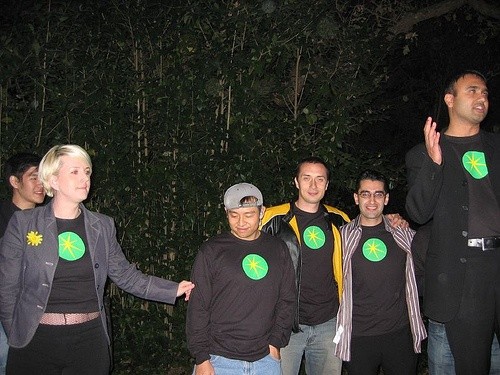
[340, 170, 428, 375]
[411, 219, 500, 375]
[258, 158, 409, 375]
[186, 183, 297, 375]
[0, 145, 195, 375]
[0, 153, 46, 375]
[406, 69, 500, 375]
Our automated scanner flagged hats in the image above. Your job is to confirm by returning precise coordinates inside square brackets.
[224, 183, 263, 210]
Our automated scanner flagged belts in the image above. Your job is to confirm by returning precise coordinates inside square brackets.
[39, 310, 100, 326]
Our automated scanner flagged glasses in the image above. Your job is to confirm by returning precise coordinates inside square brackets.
[359, 192, 386, 203]
